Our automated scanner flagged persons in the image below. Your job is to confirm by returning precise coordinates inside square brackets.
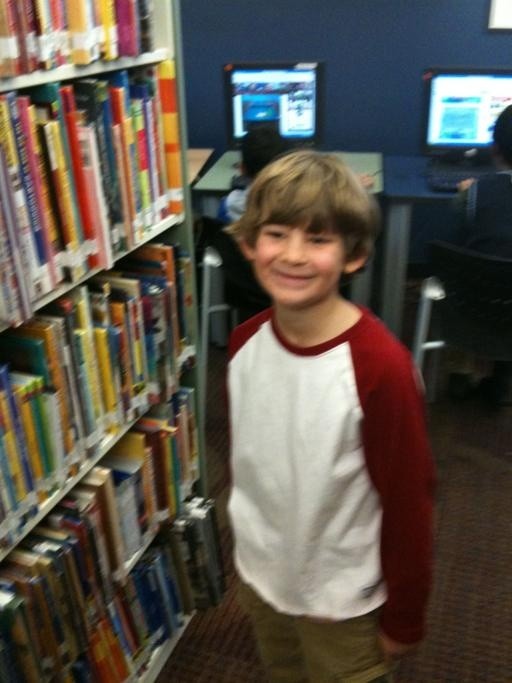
[218, 126, 373, 227]
[225, 146, 437, 683]
[413, 104, 512, 409]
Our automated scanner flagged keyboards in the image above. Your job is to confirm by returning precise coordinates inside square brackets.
[427, 174, 479, 192]
[231, 174, 248, 189]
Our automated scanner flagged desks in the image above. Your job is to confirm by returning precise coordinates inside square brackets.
[384, 147, 499, 346]
[193, 150, 384, 346]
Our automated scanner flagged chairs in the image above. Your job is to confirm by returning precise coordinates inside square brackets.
[412, 236, 511, 410]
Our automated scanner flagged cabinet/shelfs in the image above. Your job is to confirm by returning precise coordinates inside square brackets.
[1, 0, 209, 682]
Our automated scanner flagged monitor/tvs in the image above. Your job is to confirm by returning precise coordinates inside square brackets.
[417, 66, 512, 161]
[221, 59, 326, 150]
